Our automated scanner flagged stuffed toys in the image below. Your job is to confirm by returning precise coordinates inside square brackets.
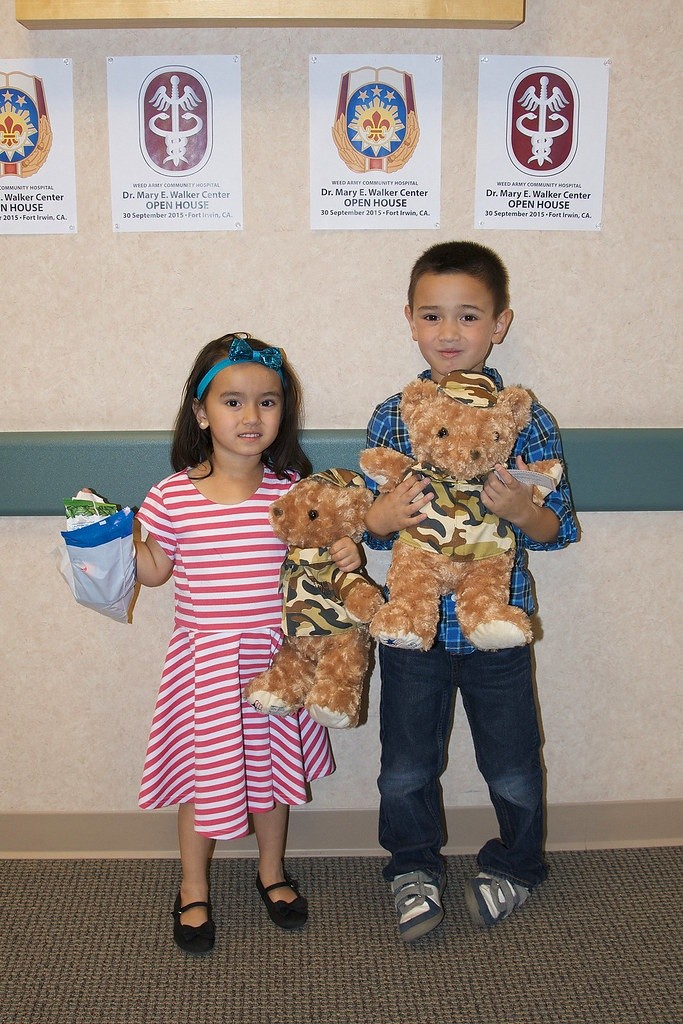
[361, 370, 563, 651]
[244, 467, 385, 729]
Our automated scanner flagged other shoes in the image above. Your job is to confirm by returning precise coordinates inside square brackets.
[170, 889, 218, 949]
[255, 866, 310, 929]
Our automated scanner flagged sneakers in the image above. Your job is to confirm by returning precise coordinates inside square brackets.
[464, 861, 531, 925]
[389, 855, 449, 940]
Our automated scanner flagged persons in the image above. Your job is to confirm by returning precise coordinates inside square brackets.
[85, 333, 362, 953]
[358, 240, 578, 939]
